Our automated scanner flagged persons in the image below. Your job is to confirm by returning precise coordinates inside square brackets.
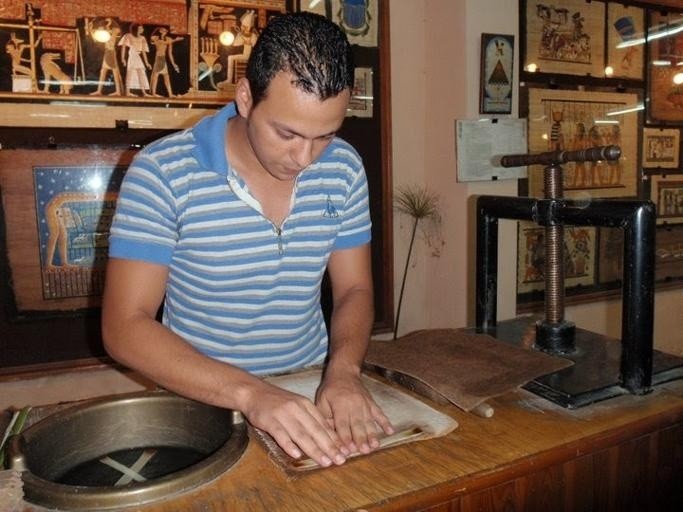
[216, 12, 257, 91]
[117, 22, 154, 99]
[4, 30, 47, 95]
[148, 25, 180, 99]
[87, 18, 121, 98]
[538, 5, 621, 190]
[99, 11, 395, 469]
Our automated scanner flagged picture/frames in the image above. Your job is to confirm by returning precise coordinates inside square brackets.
[480, 30, 516, 114]
[346, 66, 376, 121]
[519, 0, 683, 302]
[325, 0, 382, 50]
[33, 166, 134, 300]
